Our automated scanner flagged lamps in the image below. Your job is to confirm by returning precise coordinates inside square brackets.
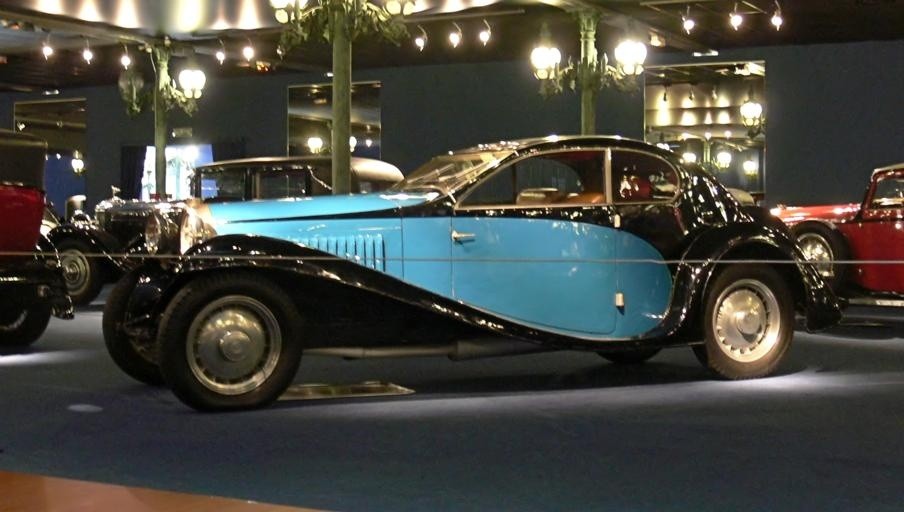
[0, 0, 783, 100]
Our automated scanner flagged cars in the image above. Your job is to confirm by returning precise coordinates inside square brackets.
[769, 159, 904, 301]
[44, 149, 408, 309]
[97, 131, 849, 414]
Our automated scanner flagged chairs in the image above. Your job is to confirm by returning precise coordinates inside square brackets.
[556, 191, 605, 204]
[515, 187, 557, 203]
